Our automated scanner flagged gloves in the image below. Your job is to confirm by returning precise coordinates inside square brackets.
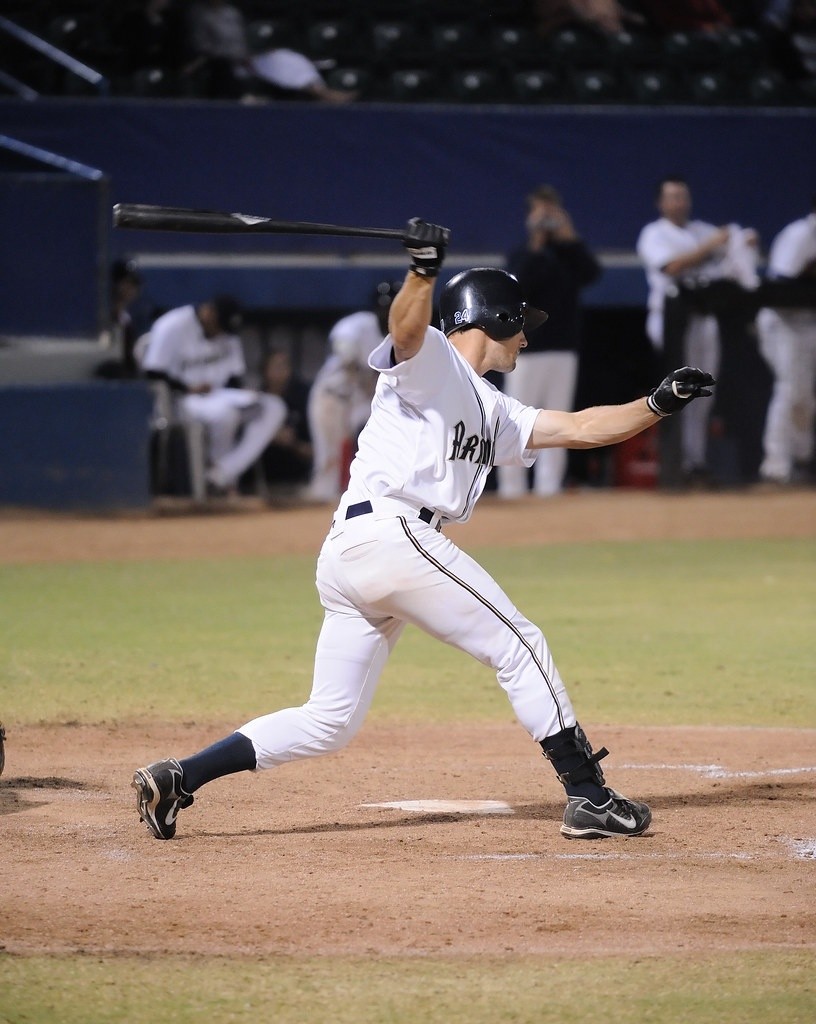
[646, 367, 716, 416]
[401, 216, 451, 276]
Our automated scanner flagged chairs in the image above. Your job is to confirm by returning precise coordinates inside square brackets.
[0, 0, 816, 108]
[144, 372, 266, 504]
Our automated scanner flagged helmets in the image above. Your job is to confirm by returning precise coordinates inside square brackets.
[112, 257, 145, 285]
[440, 267, 548, 341]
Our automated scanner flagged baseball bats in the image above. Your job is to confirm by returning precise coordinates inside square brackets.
[112, 201, 452, 246]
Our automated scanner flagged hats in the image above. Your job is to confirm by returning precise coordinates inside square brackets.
[217, 293, 243, 335]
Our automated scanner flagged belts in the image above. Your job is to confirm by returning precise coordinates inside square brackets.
[345, 500, 442, 533]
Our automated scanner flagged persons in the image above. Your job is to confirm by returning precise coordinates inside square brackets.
[296, 279, 402, 505]
[176, 0, 351, 104]
[132, 291, 287, 496]
[496, 186, 599, 500]
[257, 348, 314, 483]
[638, 175, 816, 486]
[568, 0, 816, 81]
[130, 216, 716, 841]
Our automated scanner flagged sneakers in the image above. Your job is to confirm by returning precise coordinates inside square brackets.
[131, 757, 194, 840]
[559, 787, 652, 837]
